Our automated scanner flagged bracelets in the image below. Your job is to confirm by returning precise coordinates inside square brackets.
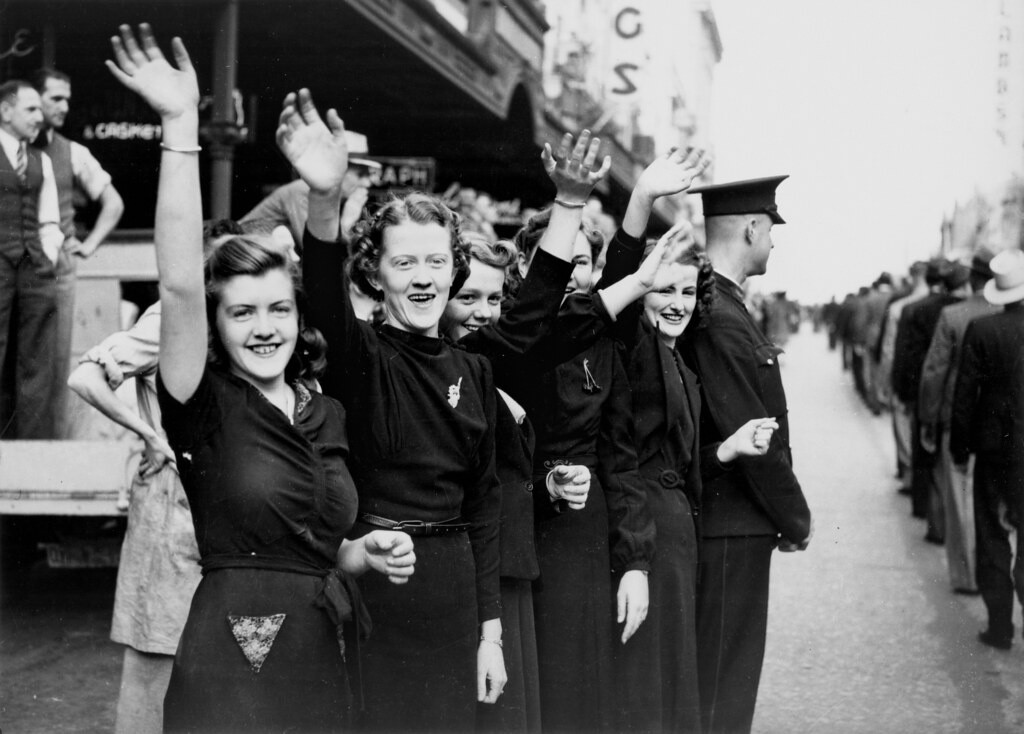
[479, 635, 503, 647]
[554, 198, 586, 209]
[159, 142, 202, 153]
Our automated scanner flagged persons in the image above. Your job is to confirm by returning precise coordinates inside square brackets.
[236, 85, 813, 734]
[30, 70, 124, 435]
[68, 217, 299, 734]
[106, 22, 417, 734]
[823, 252, 1023, 648]
[0, 81, 63, 436]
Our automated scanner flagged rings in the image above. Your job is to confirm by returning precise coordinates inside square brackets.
[500, 689, 504, 694]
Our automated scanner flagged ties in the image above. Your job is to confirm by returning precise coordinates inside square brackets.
[16, 142, 27, 184]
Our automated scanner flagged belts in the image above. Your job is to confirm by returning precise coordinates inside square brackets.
[358, 512, 469, 535]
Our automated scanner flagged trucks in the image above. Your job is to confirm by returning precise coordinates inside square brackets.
[0, 231, 160, 591]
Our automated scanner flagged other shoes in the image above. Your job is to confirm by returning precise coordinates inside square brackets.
[979, 629, 1013, 650]
[898, 487, 911, 494]
[924, 534, 944, 544]
[953, 582, 979, 594]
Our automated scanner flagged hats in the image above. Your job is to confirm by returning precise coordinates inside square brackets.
[687, 175, 791, 224]
[984, 249, 1024, 305]
